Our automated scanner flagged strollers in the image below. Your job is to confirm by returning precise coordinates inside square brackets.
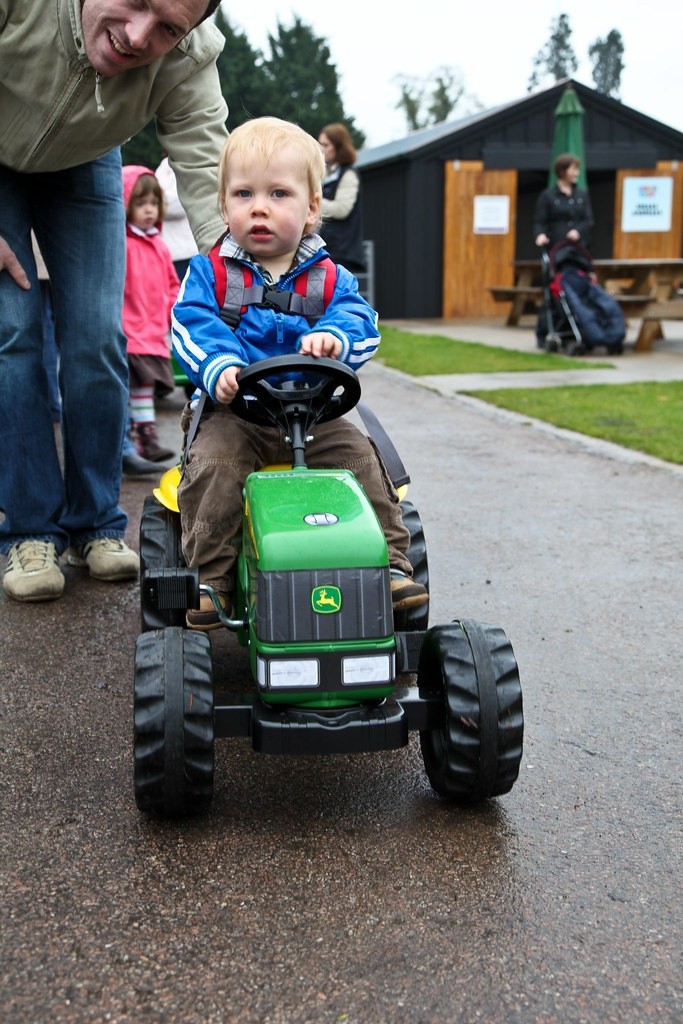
[533, 237, 627, 358]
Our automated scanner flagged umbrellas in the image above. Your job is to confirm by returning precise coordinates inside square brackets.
[549, 83, 586, 196]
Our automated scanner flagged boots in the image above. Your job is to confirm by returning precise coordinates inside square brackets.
[130, 421, 174, 462]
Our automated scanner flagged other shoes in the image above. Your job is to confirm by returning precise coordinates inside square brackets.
[123, 455, 166, 475]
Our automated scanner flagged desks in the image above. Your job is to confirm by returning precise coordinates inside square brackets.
[505, 259, 683, 352]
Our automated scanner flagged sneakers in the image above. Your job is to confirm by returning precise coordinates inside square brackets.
[390, 568, 429, 610]
[186, 589, 235, 630]
[3, 539, 65, 602]
[68, 536, 140, 581]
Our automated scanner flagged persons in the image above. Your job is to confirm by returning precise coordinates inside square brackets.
[0, 0, 428, 627]
[534, 153, 594, 348]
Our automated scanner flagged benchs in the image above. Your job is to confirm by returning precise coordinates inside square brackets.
[489, 287, 653, 302]
